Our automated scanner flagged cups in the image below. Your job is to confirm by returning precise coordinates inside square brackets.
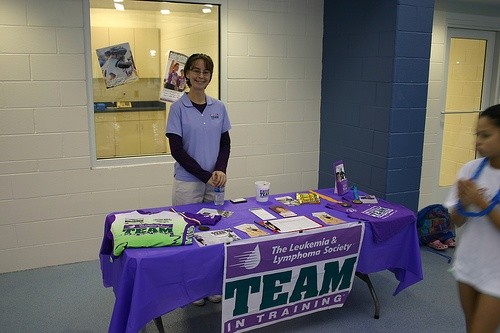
[255, 181, 271, 202]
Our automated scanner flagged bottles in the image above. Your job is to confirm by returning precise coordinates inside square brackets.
[214, 185, 225, 205]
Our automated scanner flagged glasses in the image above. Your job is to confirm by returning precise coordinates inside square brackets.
[190, 69, 210, 76]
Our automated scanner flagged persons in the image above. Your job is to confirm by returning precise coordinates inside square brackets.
[99, 46, 133, 80]
[166, 53, 232, 305]
[445, 104, 500, 333]
[166, 59, 187, 92]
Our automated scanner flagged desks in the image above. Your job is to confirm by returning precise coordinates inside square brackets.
[99, 187, 424, 333]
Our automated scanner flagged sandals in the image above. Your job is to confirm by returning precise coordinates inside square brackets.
[428, 237, 457, 250]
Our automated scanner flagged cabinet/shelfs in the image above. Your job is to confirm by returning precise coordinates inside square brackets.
[93, 109, 166, 160]
[91, 26, 167, 79]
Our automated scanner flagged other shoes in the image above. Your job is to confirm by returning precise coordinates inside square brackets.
[194, 299, 205, 306]
[209, 296, 221, 303]
